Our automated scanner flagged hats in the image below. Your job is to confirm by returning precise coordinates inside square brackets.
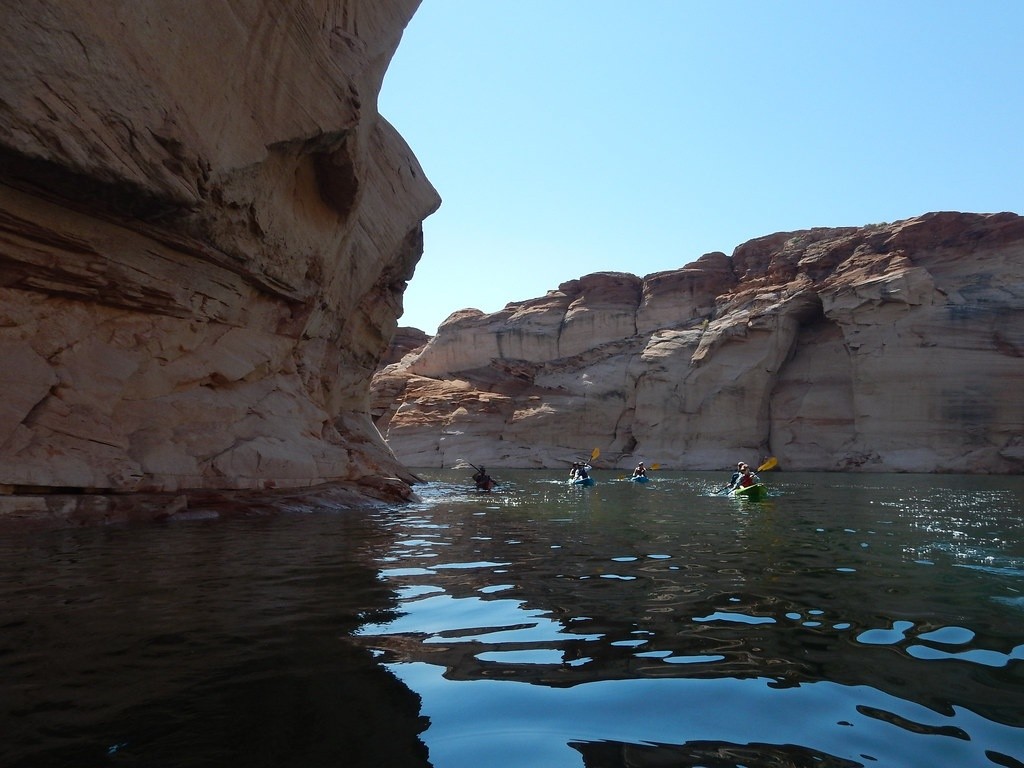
[639, 462, 643, 465]
[740, 464, 749, 471]
[738, 462, 744, 465]
[574, 462, 578, 465]
[579, 462, 584, 465]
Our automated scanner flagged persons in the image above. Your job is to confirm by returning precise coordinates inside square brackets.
[633, 461, 647, 477]
[731, 462, 745, 485]
[576, 462, 592, 479]
[734, 464, 760, 489]
[473, 466, 497, 491]
[570, 462, 578, 478]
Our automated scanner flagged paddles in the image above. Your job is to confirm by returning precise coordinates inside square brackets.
[726, 456, 778, 495]
[574, 447, 601, 482]
[461, 455, 501, 487]
[714, 465, 760, 494]
[618, 463, 660, 480]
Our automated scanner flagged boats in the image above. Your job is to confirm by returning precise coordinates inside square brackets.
[627, 475, 649, 484]
[724, 482, 769, 500]
[567, 476, 596, 487]
